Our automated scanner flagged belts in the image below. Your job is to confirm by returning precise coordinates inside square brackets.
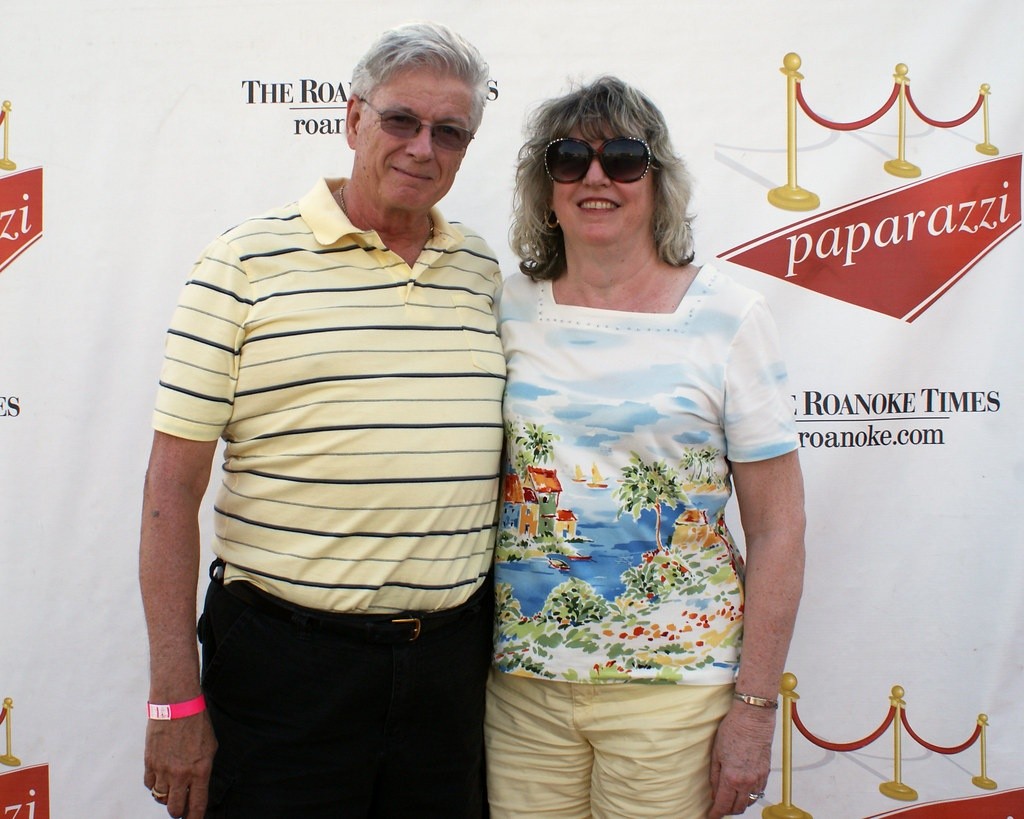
[217, 563, 490, 642]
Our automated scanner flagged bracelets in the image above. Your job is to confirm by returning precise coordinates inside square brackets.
[732, 692, 778, 708]
[145, 693, 208, 721]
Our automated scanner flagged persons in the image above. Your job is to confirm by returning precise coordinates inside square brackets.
[494, 73, 809, 819]
[136, 16, 502, 818]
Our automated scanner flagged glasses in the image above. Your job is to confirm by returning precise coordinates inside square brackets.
[544, 136, 655, 184]
[360, 98, 475, 152]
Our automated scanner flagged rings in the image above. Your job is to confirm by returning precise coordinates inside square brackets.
[750, 791, 766, 800]
[151, 787, 167, 803]
